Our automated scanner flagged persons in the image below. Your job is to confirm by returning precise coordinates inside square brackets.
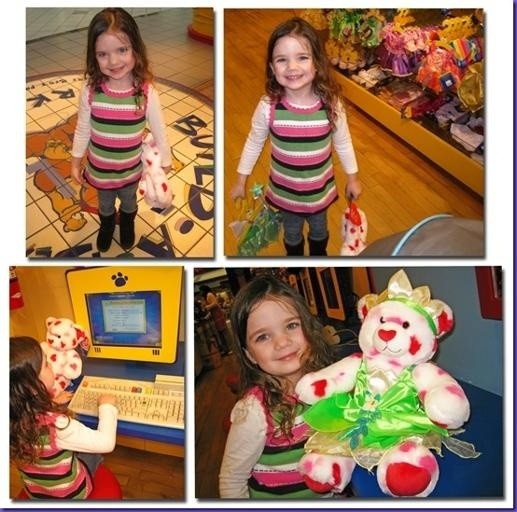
[217, 275, 351, 500]
[198, 284, 234, 356]
[9, 335, 119, 500]
[293, 269, 471, 499]
[69, 7, 172, 252]
[231, 16, 361, 256]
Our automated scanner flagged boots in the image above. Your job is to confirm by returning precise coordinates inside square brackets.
[284, 236, 304, 257]
[120, 207, 138, 249]
[96, 209, 116, 252]
[307, 231, 328, 257]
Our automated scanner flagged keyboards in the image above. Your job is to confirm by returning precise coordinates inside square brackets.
[67, 375, 184, 430]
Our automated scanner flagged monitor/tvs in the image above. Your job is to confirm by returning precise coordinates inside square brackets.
[65, 266, 184, 364]
